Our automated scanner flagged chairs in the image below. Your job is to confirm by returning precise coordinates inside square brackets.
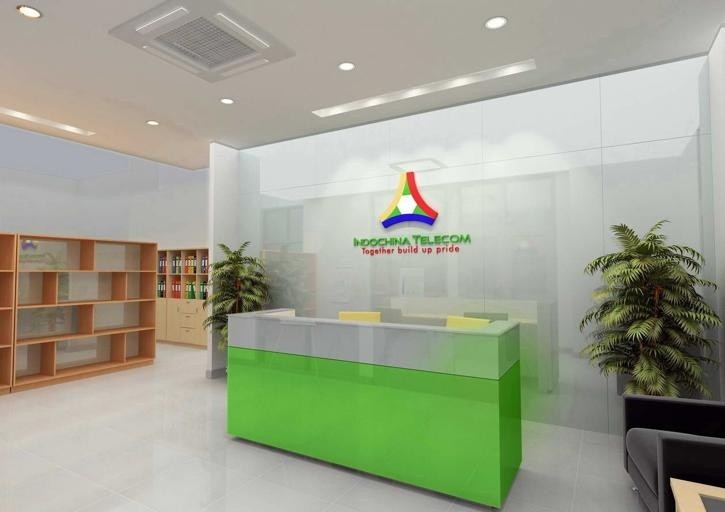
[621, 392, 725, 511]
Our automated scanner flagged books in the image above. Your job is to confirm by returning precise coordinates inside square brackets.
[157, 256, 210, 299]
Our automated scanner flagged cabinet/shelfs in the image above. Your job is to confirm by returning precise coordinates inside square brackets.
[0, 231, 209, 396]
